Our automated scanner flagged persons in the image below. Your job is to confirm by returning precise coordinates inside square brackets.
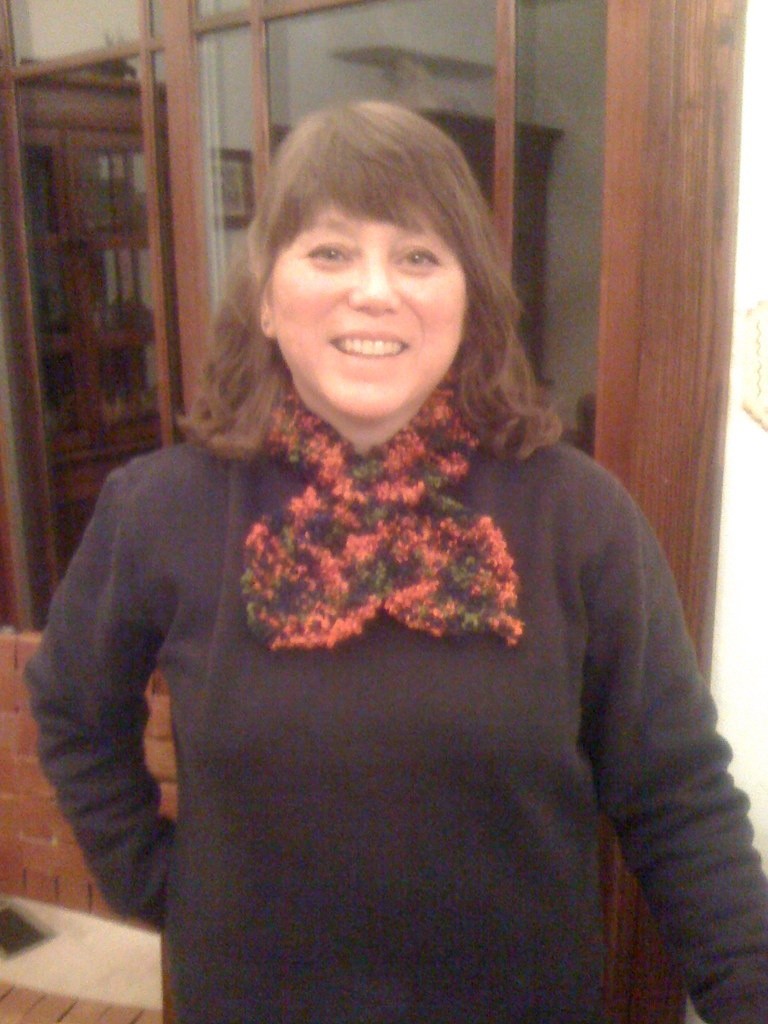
[21, 102, 768, 1024]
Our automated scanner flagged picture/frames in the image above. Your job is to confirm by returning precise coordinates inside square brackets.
[211, 144, 257, 227]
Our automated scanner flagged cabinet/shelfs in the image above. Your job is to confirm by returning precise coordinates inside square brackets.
[408, 111, 565, 389]
[19, 73, 184, 606]
